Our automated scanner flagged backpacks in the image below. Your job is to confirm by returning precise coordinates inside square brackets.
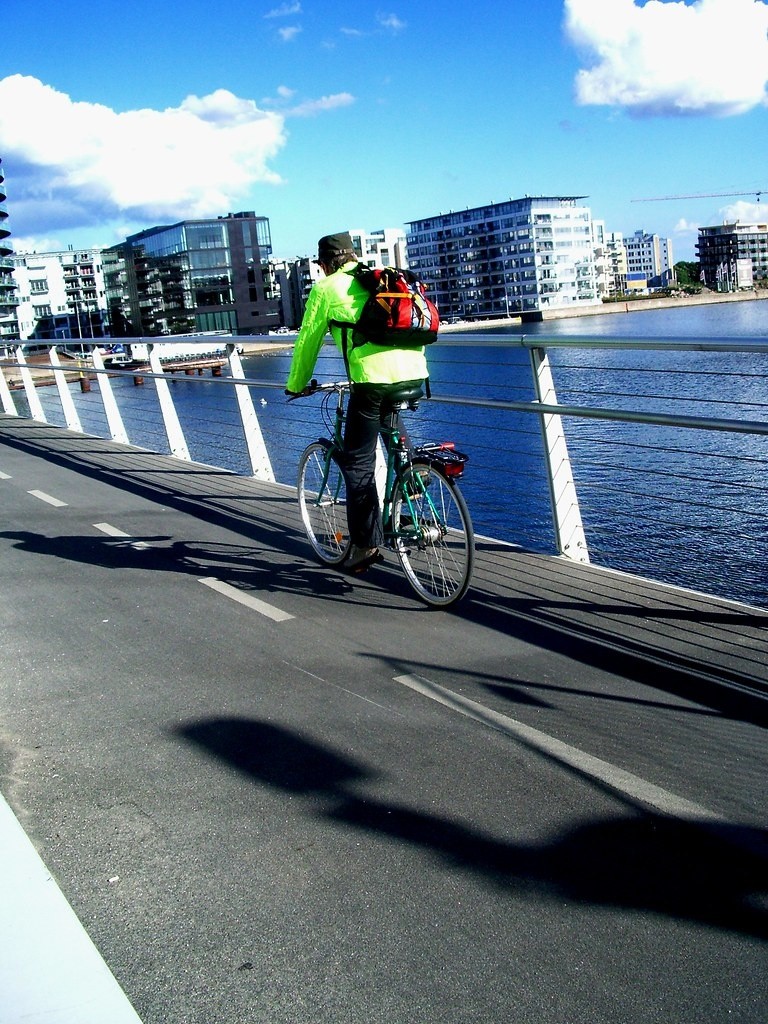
[330, 263, 440, 348]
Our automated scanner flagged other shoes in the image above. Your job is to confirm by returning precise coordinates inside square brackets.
[343, 546, 385, 571]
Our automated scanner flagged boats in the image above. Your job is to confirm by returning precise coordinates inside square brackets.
[72, 330, 246, 367]
[269, 326, 299, 336]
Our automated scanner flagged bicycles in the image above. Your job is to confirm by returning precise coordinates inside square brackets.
[285, 381, 475, 609]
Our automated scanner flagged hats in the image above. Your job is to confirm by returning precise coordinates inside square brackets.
[311, 231, 354, 263]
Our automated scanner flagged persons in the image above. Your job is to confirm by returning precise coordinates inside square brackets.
[286, 232, 430, 569]
